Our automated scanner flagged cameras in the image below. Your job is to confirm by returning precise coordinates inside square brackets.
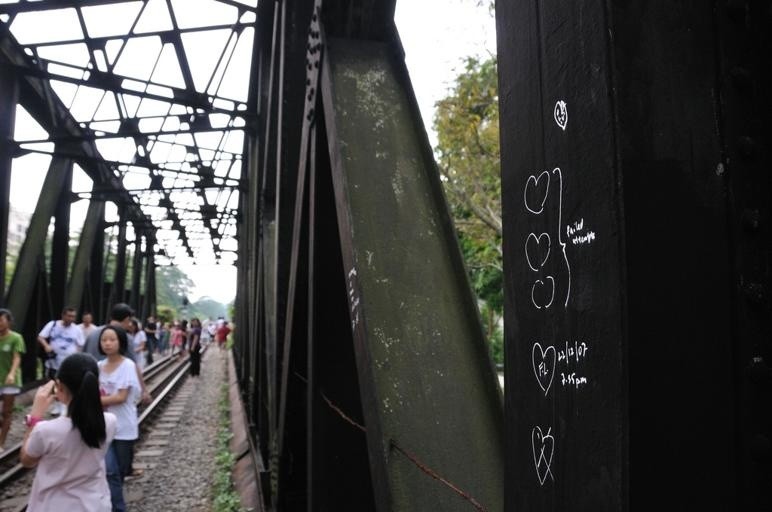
[52, 385, 62, 398]
[46, 351, 56, 360]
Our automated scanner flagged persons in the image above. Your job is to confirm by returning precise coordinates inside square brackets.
[201, 319, 233, 354]
[21, 304, 150, 512]
[144, 314, 202, 377]
[0, 308, 27, 454]
